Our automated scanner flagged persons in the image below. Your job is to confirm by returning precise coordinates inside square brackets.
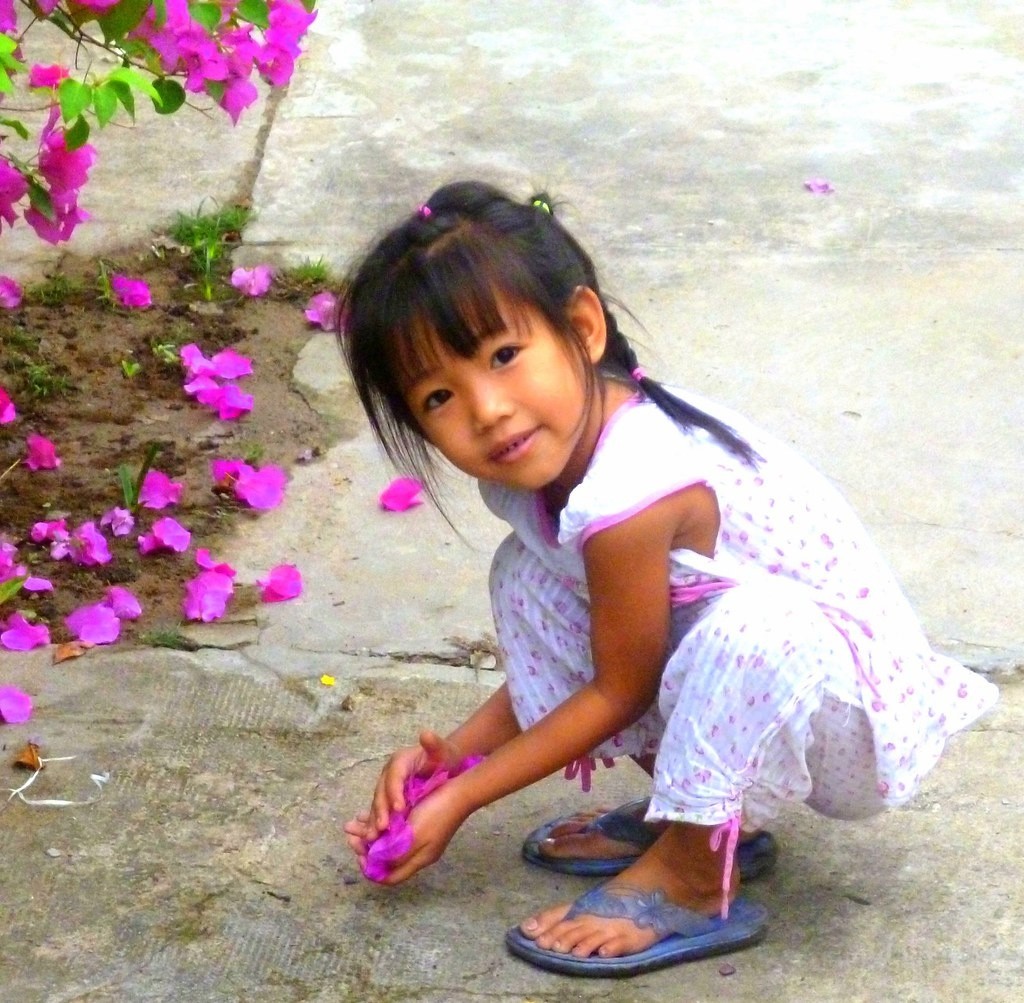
[330, 180, 1000, 978]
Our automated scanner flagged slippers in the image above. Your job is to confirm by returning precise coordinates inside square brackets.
[523, 797, 781, 878]
[502, 879, 771, 980]
